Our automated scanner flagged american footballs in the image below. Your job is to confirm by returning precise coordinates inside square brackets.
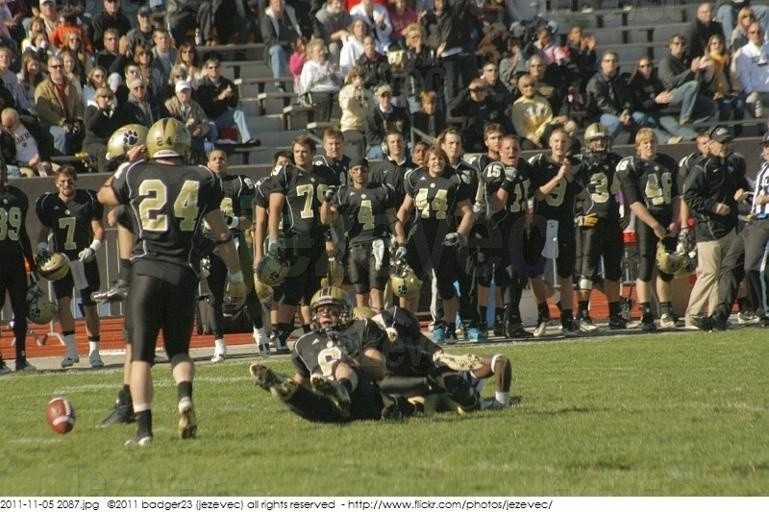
[48, 397, 75, 433]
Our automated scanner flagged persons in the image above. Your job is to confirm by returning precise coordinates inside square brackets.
[35, 168, 106, 371]
[97, 117, 245, 448]
[1, 161, 36, 372]
[255, 1, 768, 351]
[198, 149, 269, 364]
[94, 124, 167, 431]
[350, 307, 510, 414]
[1, 1, 255, 178]
[249, 286, 388, 422]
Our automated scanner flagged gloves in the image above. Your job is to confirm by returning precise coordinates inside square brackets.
[574, 212, 599, 227]
[394, 243, 408, 261]
[78, 247, 96, 264]
[442, 232, 463, 254]
[618, 215, 631, 231]
[268, 240, 284, 254]
[325, 184, 339, 203]
[504, 167, 519, 183]
[36, 248, 51, 263]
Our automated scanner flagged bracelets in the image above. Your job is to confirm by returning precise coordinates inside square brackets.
[229, 217, 238, 230]
[89, 240, 102, 253]
[227, 270, 243, 283]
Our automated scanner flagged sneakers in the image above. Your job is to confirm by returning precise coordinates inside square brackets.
[0, 277, 292, 373]
[95, 392, 133, 426]
[178, 396, 197, 439]
[122, 432, 152, 447]
[249, 362, 483, 420]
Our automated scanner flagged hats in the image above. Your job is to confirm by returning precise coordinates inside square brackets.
[176, 81, 191, 93]
[373, 83, 392, 96]
[348, 157, 369, 170]
[708, 123, 734, 144]
[139, 6, 152, 16]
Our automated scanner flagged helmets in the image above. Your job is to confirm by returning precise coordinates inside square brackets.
[583, 121, 612, 143]
[146, 117, 192, 161]
[37, 252, 70, 281]
[654, 235, 685, 274]
[255, 255, 422, 320]
[103, 124, 149, 172]
